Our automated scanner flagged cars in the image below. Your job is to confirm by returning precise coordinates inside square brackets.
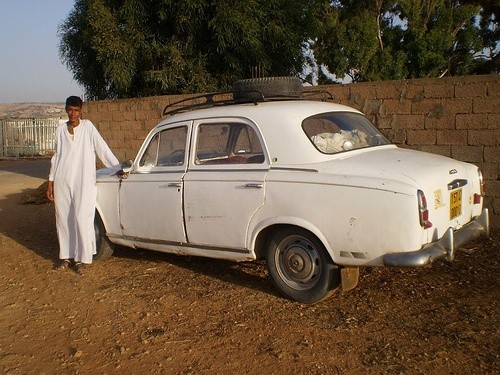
[93, 88, 491, 306]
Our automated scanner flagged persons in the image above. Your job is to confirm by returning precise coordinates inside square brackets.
[47, 96, 129, 276]
